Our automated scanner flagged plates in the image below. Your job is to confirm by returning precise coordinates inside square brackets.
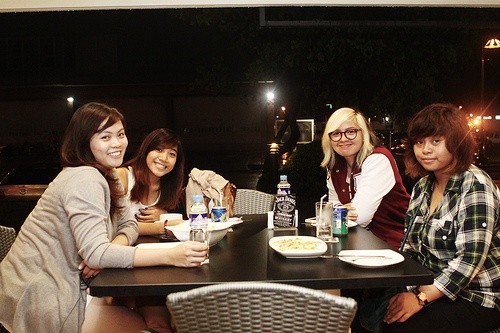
[338, 249, 405, 268]
[268, 236, 328, 259]
[347, 219, 358, 228]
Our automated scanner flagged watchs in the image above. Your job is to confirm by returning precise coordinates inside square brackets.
[412, 288, 429, 305]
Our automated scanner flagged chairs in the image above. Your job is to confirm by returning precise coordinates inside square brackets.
[233, 188, 274, 214]
[0, 226, 15, 262]
[168, 282, 357, 333]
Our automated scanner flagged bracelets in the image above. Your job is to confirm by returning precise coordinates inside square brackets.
[164, 220, 168, 236]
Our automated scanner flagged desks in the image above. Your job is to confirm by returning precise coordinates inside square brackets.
[88, 212, 434, 298]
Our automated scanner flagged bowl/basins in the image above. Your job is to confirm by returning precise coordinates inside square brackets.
[164, 220, 243, 248]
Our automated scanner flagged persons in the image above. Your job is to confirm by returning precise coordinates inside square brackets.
[320, 108, 411, 250]
[349, 101, 500, 333]
[0, 103, 208, 333]
[113, 128, 184, 235]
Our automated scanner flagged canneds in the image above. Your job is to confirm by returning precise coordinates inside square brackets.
[210, 207, 228, 223]
[331, 207, 348, 236]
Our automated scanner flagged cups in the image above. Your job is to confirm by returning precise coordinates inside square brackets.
[314, 202, 333, 239]
[159, 213, 183, 239]
[214, 197, 230, 221]
[189, 227, 210, 265]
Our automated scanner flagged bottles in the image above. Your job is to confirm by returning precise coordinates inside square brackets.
[189, 195, 209, 229]
[277, 175, 291, 195]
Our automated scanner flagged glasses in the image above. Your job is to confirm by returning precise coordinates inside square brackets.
[328, 127, 362, 142]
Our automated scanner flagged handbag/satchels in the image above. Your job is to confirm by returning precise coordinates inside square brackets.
[186, 169, 236, 218]
